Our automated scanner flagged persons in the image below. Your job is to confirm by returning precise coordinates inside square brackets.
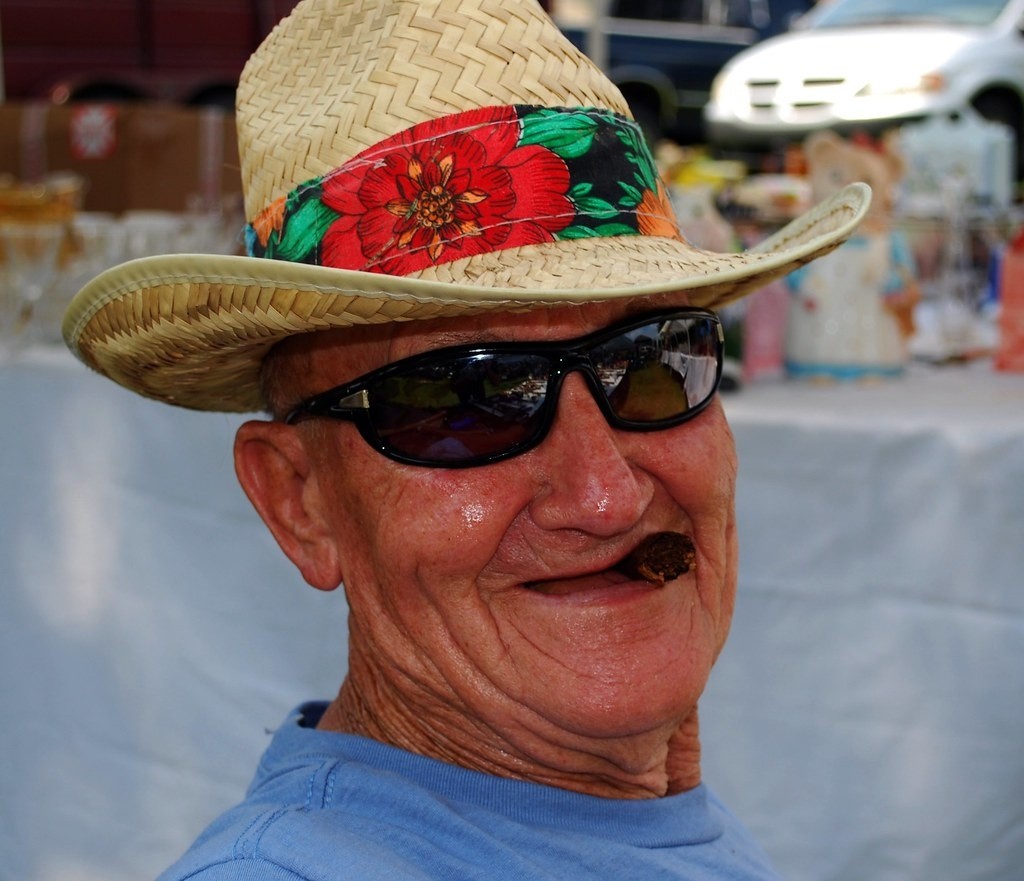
[656, 128, 919, 386]
[63, 0, 874, 881]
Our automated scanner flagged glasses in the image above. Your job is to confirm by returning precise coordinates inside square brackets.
[283, 306, 725, 467]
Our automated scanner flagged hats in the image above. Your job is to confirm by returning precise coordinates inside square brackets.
[63, 0, 873, 413]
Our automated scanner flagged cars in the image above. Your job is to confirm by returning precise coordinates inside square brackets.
[704, 0, 1024, 198]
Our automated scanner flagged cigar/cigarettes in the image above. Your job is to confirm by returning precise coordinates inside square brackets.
[633, 531, 696, 582]
